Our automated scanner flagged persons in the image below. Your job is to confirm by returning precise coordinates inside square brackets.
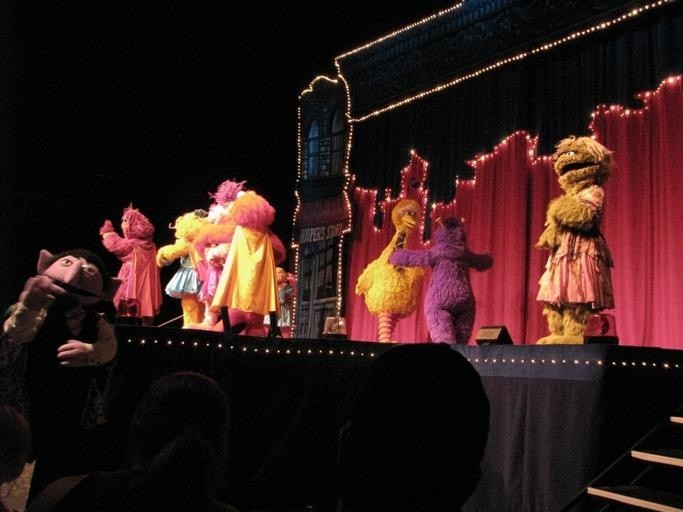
[0, 404, 32, 512]
[22, 369, 246, 512]
[300, 342, 491, 511]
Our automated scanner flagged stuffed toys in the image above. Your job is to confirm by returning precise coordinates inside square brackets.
[531, 134, 617, 345]
[0, 246, 119, 512]
[353, 199, 427, 343]
[100, 177, 295, 338]
[387, 215, 494, 345]
